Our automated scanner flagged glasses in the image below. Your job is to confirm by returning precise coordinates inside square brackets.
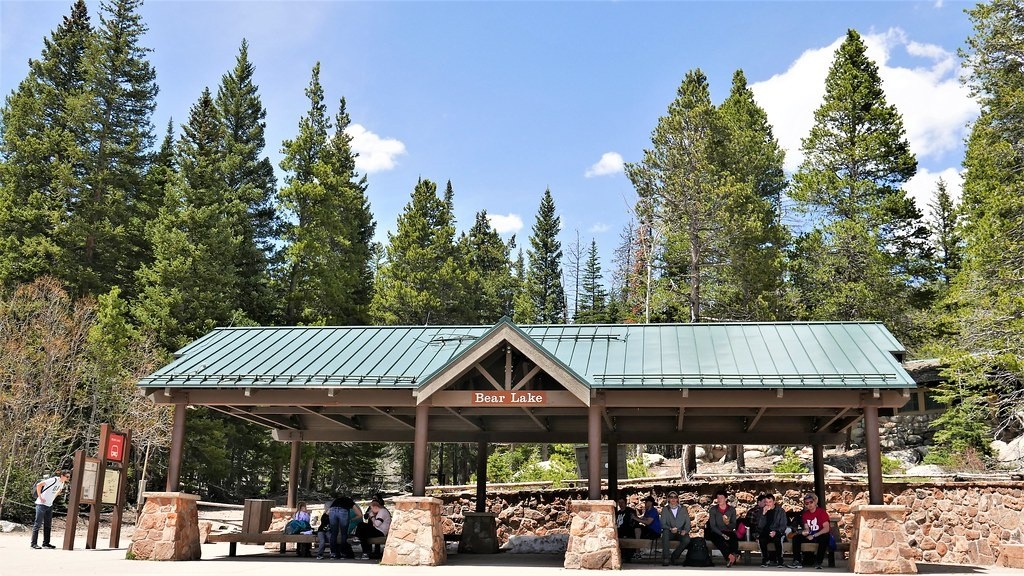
[805, 500, 814, 505]
[63, 475, 70, 478]
[669, 496, 678, 499]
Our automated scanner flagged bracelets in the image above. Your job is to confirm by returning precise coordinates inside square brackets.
[813, 534, 815, 538]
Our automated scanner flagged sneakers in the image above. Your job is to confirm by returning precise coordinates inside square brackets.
[786, 559, 803, 568]
[42, 544, 56, 549]
[760, 557, 771, 567]
[812, 562, 823, 569]
[31, 544, 42, 549]
[631, 553, 642, 560]
[772, 556, 785, 568]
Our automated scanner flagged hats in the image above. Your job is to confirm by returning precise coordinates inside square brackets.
[667, 491, 678, 498]
[640, 496, 658, 506]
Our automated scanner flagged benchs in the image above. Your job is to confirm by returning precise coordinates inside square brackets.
[368, 534, 461, 555]
[207, 532, 354, 558]
[619, 537, 850, 564]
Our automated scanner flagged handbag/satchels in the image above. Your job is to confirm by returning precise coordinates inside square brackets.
[703, 519, 718, 541]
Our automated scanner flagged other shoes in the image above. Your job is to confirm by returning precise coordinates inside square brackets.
[355, 553, 370, 560]
[296, 552, 302, 556]
[727, 553, 742, 567]
[339, 552, 346, 560]
[316, 555, 324, 560]
[329, 552, 336, 560]
[662, 558, 679, 566]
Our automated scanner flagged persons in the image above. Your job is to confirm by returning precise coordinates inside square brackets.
[660, 491, 691, 566]
[615, 498, 636, 563]
[709, 491, 740, 568]
[747, 494, 787, 568]
[632, 496, 661, 559]
[786, 493, 831, 569]
[31, 470, 71, 549]
[295, 490, 392, 559]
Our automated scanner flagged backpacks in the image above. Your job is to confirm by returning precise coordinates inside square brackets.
[683, 537, 712, 567]
[32, 478, 57, 498]
